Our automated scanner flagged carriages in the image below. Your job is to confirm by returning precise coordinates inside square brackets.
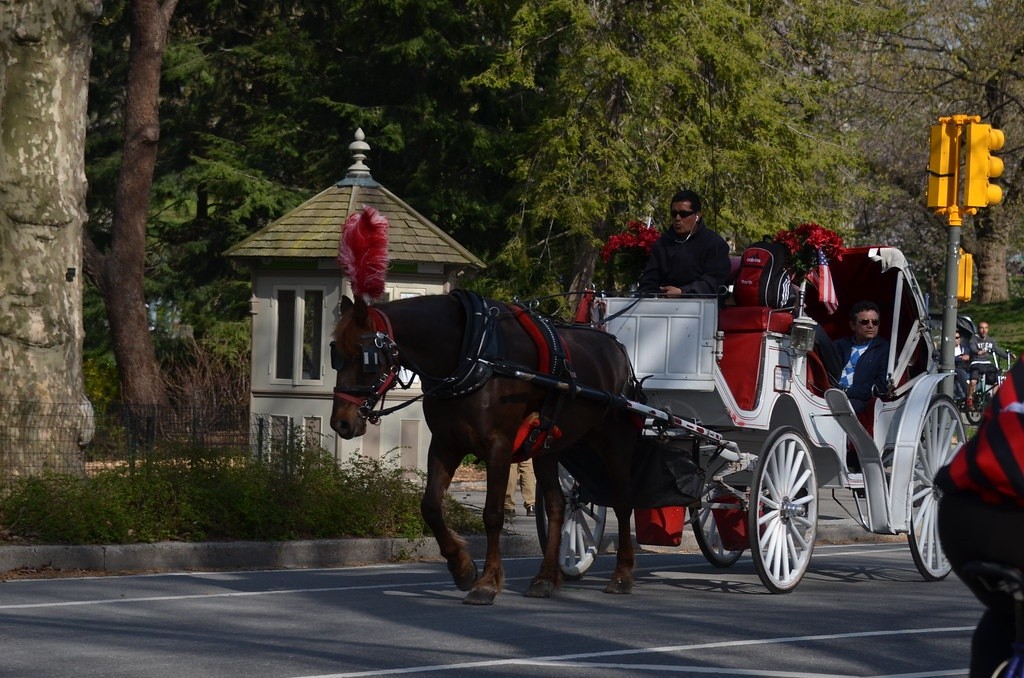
[330, 203, 968, 605]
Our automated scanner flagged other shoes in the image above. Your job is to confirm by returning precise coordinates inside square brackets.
[966, 396, 973, 409]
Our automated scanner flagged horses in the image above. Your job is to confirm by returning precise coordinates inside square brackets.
[329, 291, 636, 605]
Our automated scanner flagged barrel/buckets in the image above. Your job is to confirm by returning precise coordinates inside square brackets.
[635, 506, 686, 547]
[708, 493, 767, 550]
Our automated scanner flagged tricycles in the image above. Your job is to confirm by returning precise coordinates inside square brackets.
[928, 314, 1016, 425]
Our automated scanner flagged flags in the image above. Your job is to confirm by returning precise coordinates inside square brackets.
[803, 244, 842, 314]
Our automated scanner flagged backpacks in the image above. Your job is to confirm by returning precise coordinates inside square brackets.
[735, 234, 790, 310]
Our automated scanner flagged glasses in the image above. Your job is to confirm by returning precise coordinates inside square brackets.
[955, 336, 960, 339]
[670, 209, 696, 219]
[854, 319, 881, 326]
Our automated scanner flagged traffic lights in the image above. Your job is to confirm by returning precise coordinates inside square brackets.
[964, 123, 1004, 208]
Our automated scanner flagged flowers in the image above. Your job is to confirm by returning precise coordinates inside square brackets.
[769, 220, 846, 285]
[600, 220, 663, 297]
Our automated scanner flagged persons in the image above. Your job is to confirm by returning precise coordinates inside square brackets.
[966, 322, 1016, 408]
[933, 353, 1024, 678]
[813, 301, 898, 415]
[639, 190, 731, 299]
[954, 330, 970, 396]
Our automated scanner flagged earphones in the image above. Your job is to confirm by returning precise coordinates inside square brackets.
[696, 215, 698, 221]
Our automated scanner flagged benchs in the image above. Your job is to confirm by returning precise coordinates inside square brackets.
[717, 255, 793, 411]
